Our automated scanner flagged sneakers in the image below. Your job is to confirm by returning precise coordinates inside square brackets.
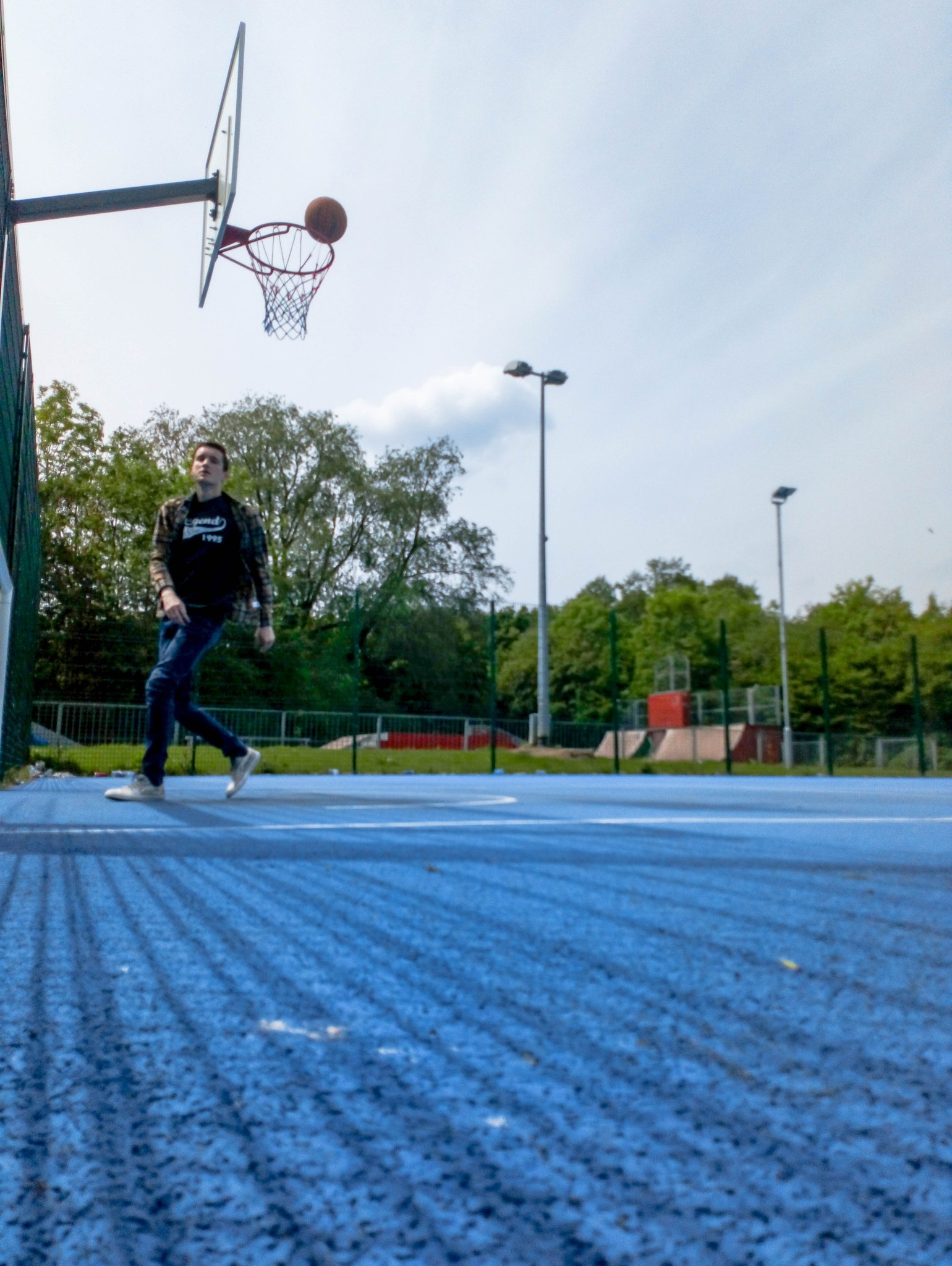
[225, 747, 261, 799]
[104, 774, 167, 800]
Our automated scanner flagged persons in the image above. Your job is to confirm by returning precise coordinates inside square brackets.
[104, 442, 276, 802]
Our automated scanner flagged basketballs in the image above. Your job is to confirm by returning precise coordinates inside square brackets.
[304, 196, 348, 245]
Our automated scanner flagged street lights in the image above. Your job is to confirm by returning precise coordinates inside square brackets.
[501, 358, 569, 746]
[770, 485, 797, 773]
